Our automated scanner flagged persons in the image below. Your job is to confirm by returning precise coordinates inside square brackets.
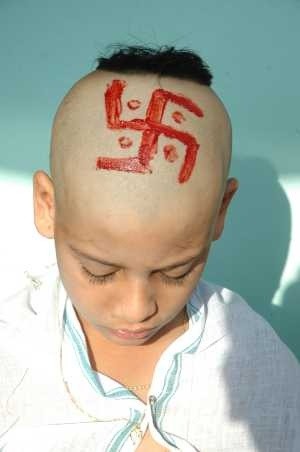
[1, 44, 298, 452]
